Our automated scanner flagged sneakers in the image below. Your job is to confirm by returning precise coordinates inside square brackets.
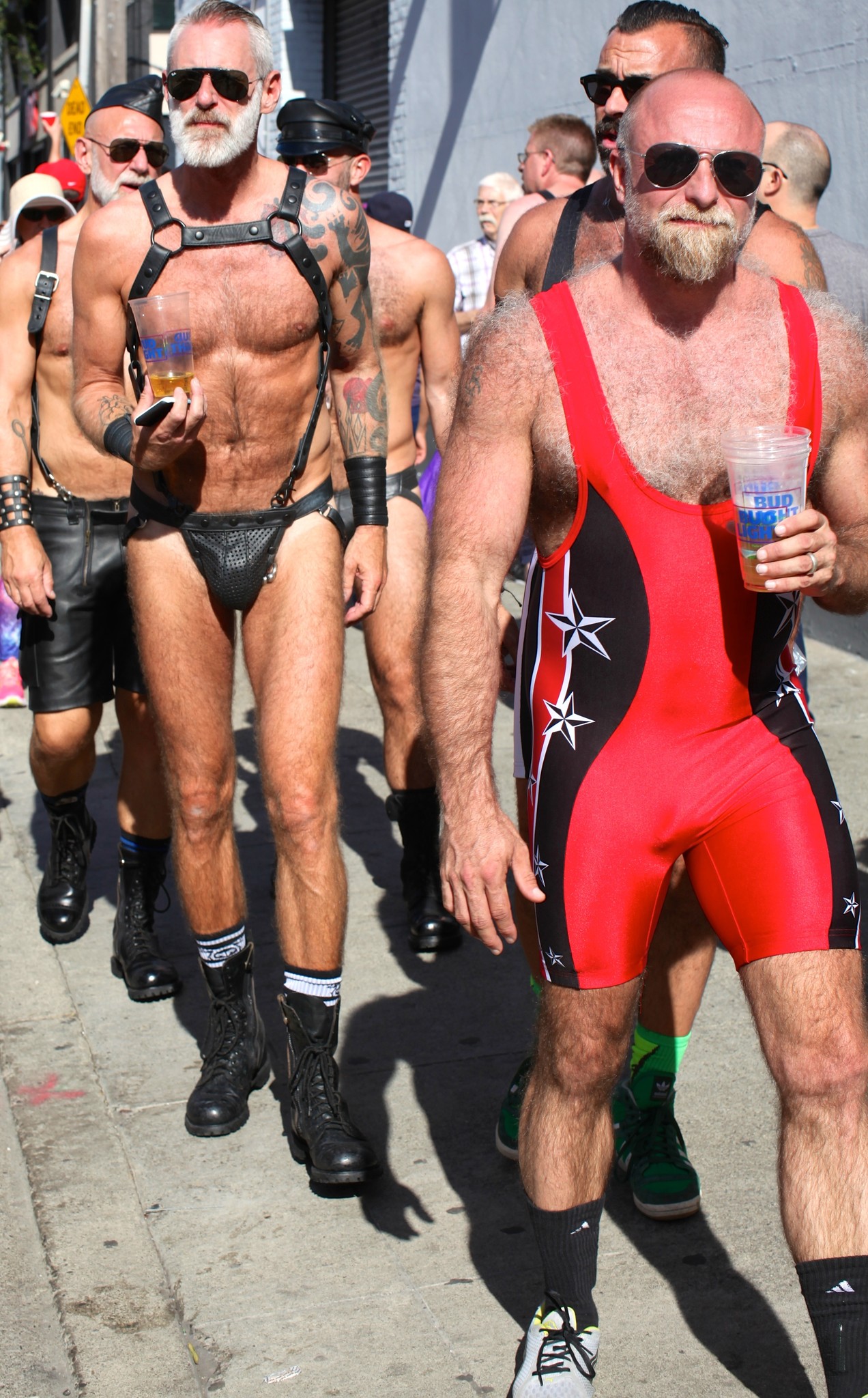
[612, 1071, 702, 1218]
[514, 1296, 601, 1398]
[1, 657, 25, 707]
[496, 1053, 537, 1158]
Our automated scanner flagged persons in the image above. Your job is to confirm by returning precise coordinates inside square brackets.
[0, 0, 867, 1221]
[424, 72, 868, 1398]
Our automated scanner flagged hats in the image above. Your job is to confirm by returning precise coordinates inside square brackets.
[275, 98, 375, 156]
[35, 159, 86, 202]
[8, 174, 77, 250]
[84, 74, 166, 138]
[365, 192, 414, 234]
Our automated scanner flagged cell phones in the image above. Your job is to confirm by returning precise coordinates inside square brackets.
[133, 398, 193, 427]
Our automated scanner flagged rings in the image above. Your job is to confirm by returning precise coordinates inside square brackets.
[805, 552, 819, 576]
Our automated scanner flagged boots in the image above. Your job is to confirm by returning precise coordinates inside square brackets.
[389, 787, 463, 950]
[277, 988, 383, 1183]
[37, 788, 100, 942]
[110, 852, 181, 1002]
[184, 942, 271, 1137]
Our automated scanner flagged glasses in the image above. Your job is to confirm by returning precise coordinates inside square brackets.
[164, 68, 263, 102]
[518, 150, 547, 163]
[23, 205, 66, 222]
[277, 153, 355, 175]
[580, 74, 651, 104]
[474, 199, 509, 208]
[86, 135, 168, 168]
[618, 143, 763, 197]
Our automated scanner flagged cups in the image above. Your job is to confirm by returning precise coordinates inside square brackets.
[721, 423, 812, 593]
[127, 289, 196, 397]
[40, 112, 56, 132]
